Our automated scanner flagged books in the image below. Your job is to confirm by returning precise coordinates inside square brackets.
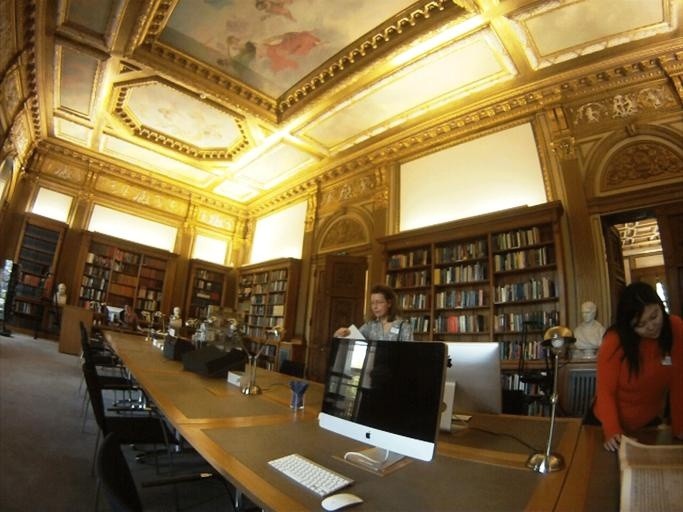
[15, 301, 32, 314]
[613, 434, 683, 512]
[190, 270, 225, 320]
[79, 241, 167, 325]
[241, 271, 288, 370]
[386, 227, 560, 416]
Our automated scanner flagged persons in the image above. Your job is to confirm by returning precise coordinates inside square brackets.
[569, 302, 606, 351]
[333, 286, 416, 341]
[173, 307, 182, 321]
[53, 283, 67, 306]
[582, 282, 683, 452]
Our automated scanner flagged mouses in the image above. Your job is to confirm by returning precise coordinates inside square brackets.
[321, 493, 363, 512]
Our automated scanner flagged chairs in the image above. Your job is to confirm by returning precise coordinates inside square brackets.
[77, 320, 240, 512]
[501, 389, 529, 414]
[278, 359, 304, 377]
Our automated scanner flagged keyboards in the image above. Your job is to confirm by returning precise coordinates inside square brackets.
[453, 413, 470, 422]
[267, 452, 355, 497]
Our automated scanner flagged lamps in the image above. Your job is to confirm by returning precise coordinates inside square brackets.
[526, 326, 576, 473]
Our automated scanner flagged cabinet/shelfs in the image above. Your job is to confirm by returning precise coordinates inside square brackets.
[4, 213, 68, 334]
[432, 212, 494, 342]
[490, 198, 567, 392]
[377, 225, 432, 341]
[234, 258, 301, 370]
[73, 231, 178, 328]
[183, 260, 234, 336]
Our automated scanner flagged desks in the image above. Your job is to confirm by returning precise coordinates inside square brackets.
[174, 395, 583, 512]
[554, 424, 683, 512]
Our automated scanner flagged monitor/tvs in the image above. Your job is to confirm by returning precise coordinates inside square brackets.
[438, 339, 504, 432]
[317, 335, 447, 473]
